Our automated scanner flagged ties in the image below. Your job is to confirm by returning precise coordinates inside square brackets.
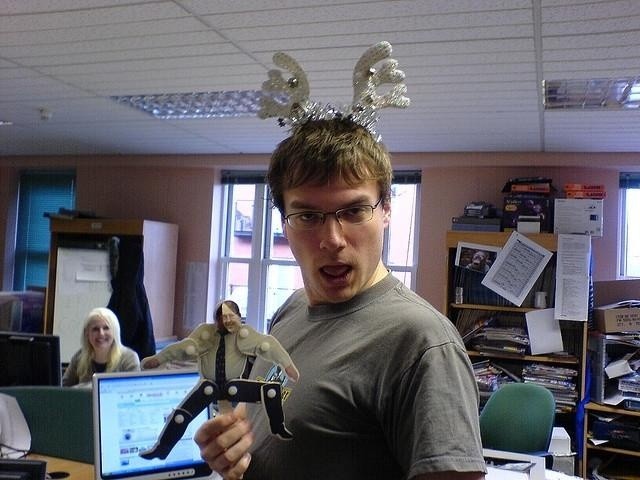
[215, 331, 226, 384]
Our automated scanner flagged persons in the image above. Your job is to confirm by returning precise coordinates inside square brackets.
[63, 308, 140, 387]
[141, 301, 298, 459]
[195, 120, 488, 480]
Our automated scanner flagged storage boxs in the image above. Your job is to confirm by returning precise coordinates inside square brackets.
[596, 301, 640, 334]
[554, 198, 603, 236]
[503, 195, 553, 233]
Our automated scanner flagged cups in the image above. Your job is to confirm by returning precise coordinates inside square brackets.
[533, 291, 548, 310]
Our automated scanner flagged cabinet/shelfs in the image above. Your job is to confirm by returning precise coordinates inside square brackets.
[583, 405, 640, 479]
[443, 231, 586, 478]
[44, 216, 179, 365]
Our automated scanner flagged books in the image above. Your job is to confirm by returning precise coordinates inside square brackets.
[472, 327, 529, 355]
[472, 360, 521, 391]
[462, 315, 496, 344]
[522, 363, 578, 414]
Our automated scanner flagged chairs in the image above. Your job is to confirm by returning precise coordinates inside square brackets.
[478, 383, 555, 453]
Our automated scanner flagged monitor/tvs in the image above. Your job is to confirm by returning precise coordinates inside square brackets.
[93, 369, 216, 480]
[0, 458, 47, 480]
[1, 332, 62, 386]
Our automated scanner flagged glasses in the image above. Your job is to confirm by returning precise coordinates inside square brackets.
[281, 196, 383, 230]
